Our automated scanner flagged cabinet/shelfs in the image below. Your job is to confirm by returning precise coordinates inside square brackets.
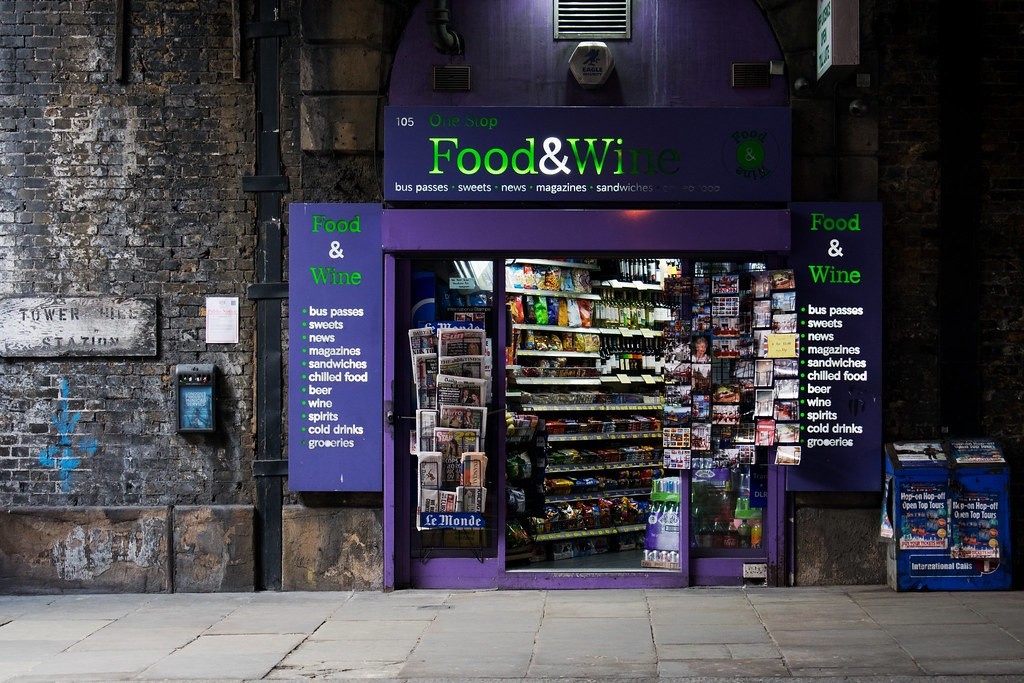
[507, 260, 667, 540]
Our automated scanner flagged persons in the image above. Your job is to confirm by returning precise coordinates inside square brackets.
[468, 343, 479, 355]
[472, 393, 479, 404]
[461, 409, 472, 429]
[462, 389, 473, 403]
[922, 444, 939, 460]
[447, 439, 463, 457]
[692, 336, 711, 362]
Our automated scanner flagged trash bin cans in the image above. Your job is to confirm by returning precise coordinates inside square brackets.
[884, 441, 1014, 593]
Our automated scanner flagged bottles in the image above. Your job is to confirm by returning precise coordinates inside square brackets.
[618, 257, 681, 285]
[738, 519, 751, 548]
[699, 518, 713, 546]
[651, 503, 680, 512]
[713, 518, 725, 547]
[726, 518, 738, 548]
[751, 520, 761, 549]
[599, 336, 665, 375]
[592, 298, 672, 330]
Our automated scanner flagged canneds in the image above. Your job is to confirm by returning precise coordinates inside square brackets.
[643, 550, 678, 563]
[652, 477, 678, 494]
